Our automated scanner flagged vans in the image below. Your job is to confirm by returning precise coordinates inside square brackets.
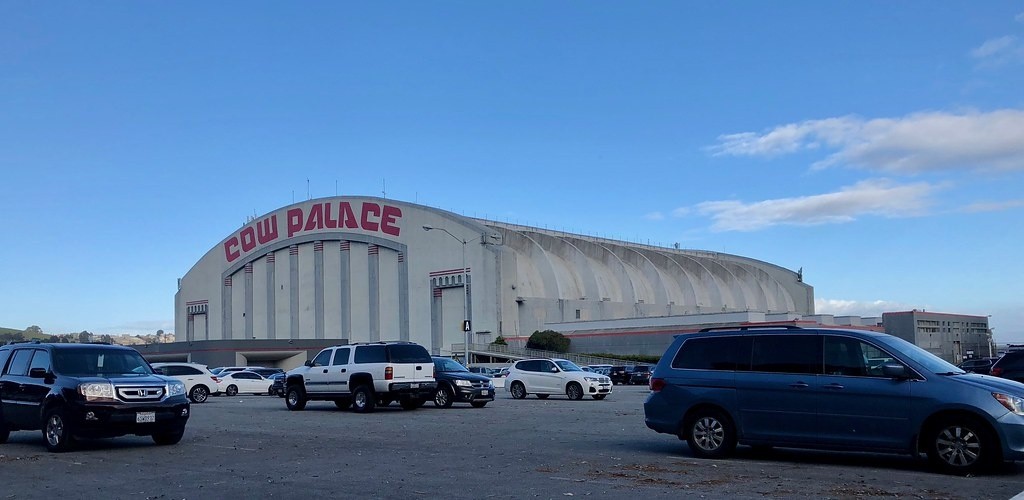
[644, 319, 1024, 473]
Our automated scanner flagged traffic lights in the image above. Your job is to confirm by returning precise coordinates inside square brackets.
[461, 319, 471, 331]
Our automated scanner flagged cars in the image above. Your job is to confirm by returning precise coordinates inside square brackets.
[273, 372, 285, 397]
[210, 370, 275, 396]
[567, 366, 610, 376]
[873, 359, 901, 369]
[376, 356, 496, 409]
[210, 366, 283, 378]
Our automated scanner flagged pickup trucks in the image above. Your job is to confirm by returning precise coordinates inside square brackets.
[468, 366, 494, 378]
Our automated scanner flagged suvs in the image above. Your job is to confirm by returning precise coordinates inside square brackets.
[865, 357, 893, 369]
[0, 339, 190, 453]
[631, 365, 655, 385]
[610, 365, 635, 385]
[132, 362, 220, 403]
[489, 368, 509, 377]
[989, 344, 1024, 383]
[282, 340, 438, 412]
[957, 357, 998, 375]
[503, 357, 613, 401]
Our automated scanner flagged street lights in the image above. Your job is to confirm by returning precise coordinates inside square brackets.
[989, 328, 994, 364]
[422, 225, 501, 370]
